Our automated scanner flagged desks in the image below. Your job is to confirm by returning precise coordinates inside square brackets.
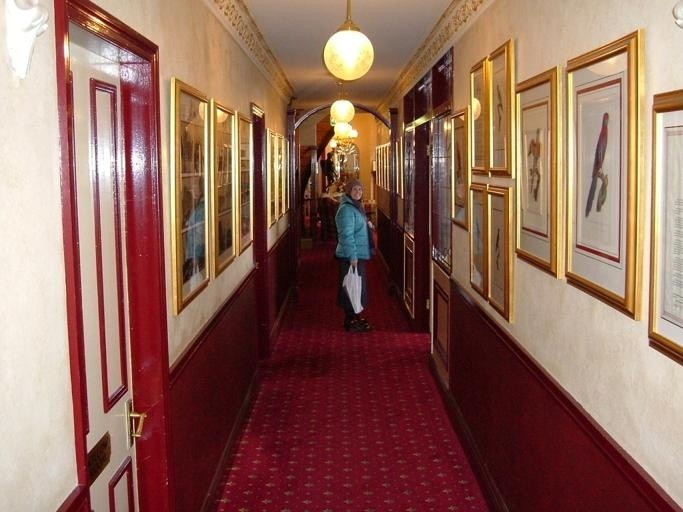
[319, 192, 343, 241]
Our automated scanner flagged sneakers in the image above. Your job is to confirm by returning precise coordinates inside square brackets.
[345, 316, 371, 332]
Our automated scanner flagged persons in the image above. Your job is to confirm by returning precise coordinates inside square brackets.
[182, 175, 205, 281]
[330, 178, 377, 335]
[323, 152, 337, 190]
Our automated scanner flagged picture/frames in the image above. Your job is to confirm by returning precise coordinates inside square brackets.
[170, 78, 290, 316]
[515, 65, 562, 280]
[562, 28, 646, 323]
[648, 88, 683, 366]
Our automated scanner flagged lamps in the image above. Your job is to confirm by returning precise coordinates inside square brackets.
[329, 82, 355, 138]
[323, 0, 374, 81]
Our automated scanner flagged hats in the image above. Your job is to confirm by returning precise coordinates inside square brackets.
[343, 179, 362, 195]
